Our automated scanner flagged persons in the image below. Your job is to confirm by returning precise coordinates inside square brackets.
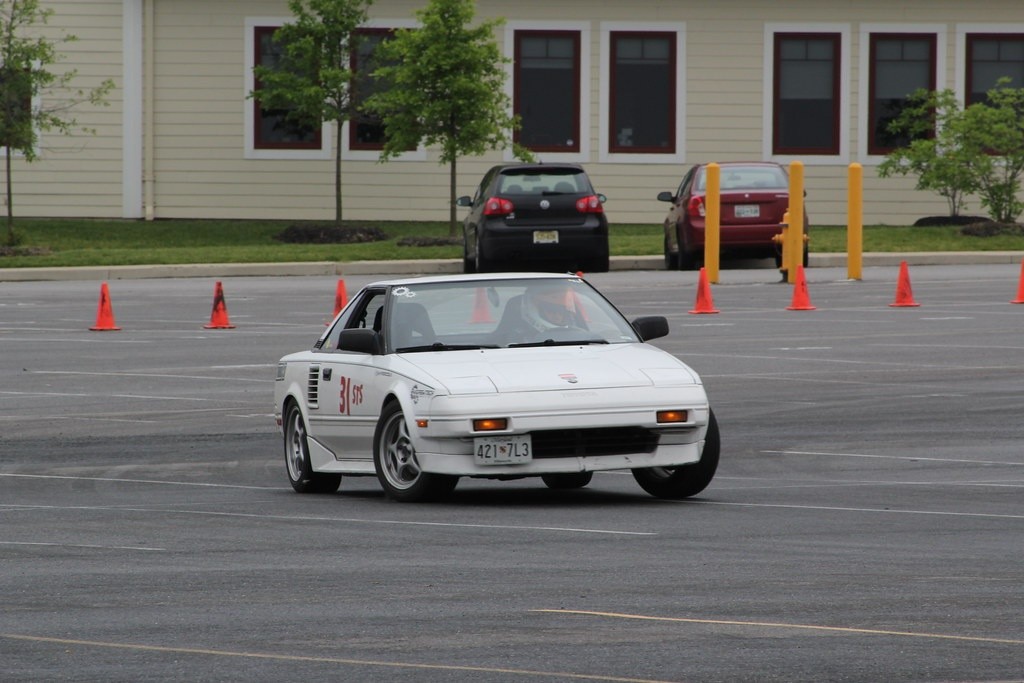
[504, 279, 585, 344]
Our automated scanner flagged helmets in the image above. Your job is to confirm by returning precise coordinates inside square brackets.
[521, 281, 576, 334]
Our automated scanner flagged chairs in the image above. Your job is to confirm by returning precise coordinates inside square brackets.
[555, 181, 575, 192]
[373, 303, 435, 344]
[495, 296, 590, 334]
[504, 185, 523, 193]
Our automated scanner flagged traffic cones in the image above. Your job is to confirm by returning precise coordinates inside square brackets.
[323, 279, 348, 326]
[201, 281, 235, 330]
[466, 286, 495, 324]
[85, 282, 123, 333]
[687, 266, 721, 314]
[887, 259, 924, 308]
[783, 266, 815, 311]
[1010, 261, 1024, 306]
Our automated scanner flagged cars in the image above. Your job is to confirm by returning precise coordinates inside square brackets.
[274, 269, 722, 505]
[657, 160, 812, 272]
[453, 159, 611, 274]
